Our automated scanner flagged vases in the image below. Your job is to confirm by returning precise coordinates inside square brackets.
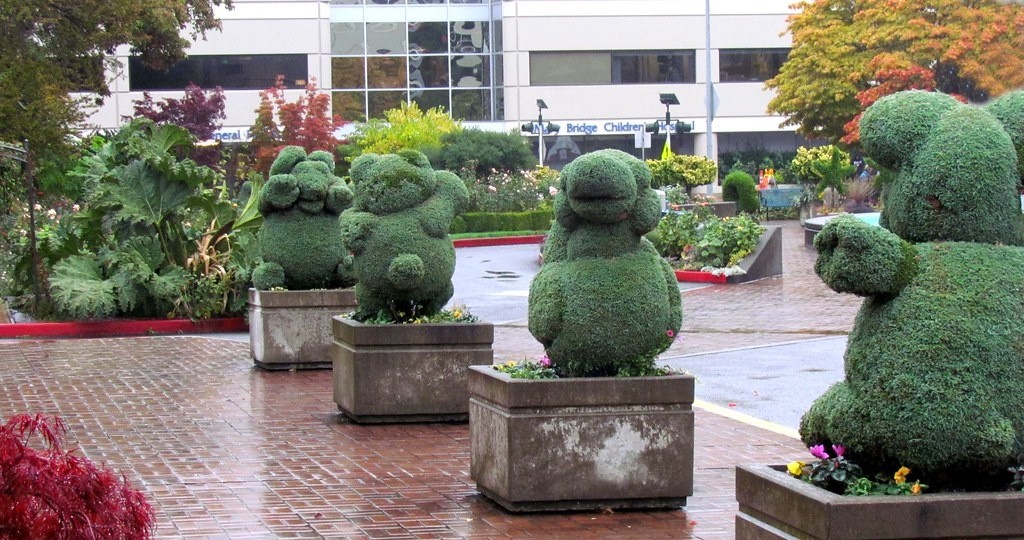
[736, 462, 1024, 540]
[333, 315, 494, 425]
[469, 365, 695, 514]
[247, 288, 360, 374]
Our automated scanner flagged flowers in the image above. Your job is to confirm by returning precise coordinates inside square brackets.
[784, 443, 1024, 497]
[251, 286, 359, 291]
[339, 299, 482, 323]
[492, 330, 682, 379]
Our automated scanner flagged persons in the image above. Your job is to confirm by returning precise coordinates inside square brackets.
[763, 168, 778, 191]
[850, 152, 881, 180]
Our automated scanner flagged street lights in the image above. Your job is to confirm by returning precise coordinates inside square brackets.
[659, 93, 680, 168]
[537, 99, 548, 169]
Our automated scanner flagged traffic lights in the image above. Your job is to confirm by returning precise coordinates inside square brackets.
[521, 123, 533, 132]
[675, 122, 691, 135]
[548, 123, 560, 133]
[645, 121, 659, 135]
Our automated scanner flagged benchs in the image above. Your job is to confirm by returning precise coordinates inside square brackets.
[759, 189, 808, 221]
